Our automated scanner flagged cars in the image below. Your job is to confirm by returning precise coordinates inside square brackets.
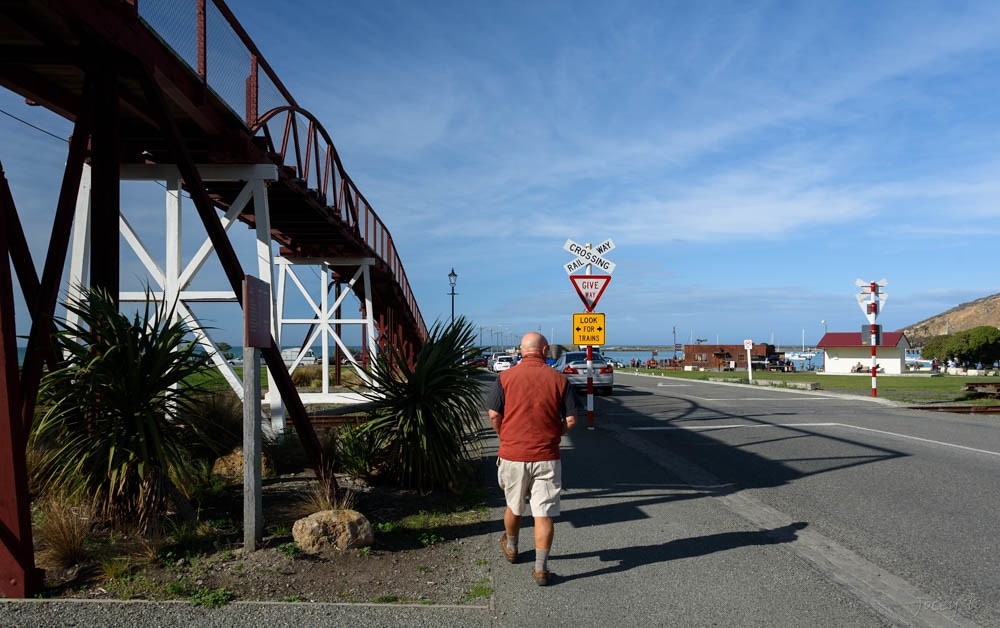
[551, 350, 613, 396]
[606, 359, 625, 369]
[469, 350, 557, 373]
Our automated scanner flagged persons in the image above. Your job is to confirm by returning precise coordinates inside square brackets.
[628, 357, 659, 369]
[769, 357, 815, 372]
[915, 357, 1000, 372]
[487, 331, 580, 588]
[876, 363, 879, 369]
[855, 362, 864, 373]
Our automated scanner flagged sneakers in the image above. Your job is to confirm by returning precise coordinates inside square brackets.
[502, 532, 519, 563]
[532, 570, 549, 586]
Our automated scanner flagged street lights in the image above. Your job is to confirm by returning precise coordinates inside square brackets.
[447, 266, 458, 334]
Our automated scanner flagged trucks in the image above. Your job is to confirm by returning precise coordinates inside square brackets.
[578, 345, 604, 356]
